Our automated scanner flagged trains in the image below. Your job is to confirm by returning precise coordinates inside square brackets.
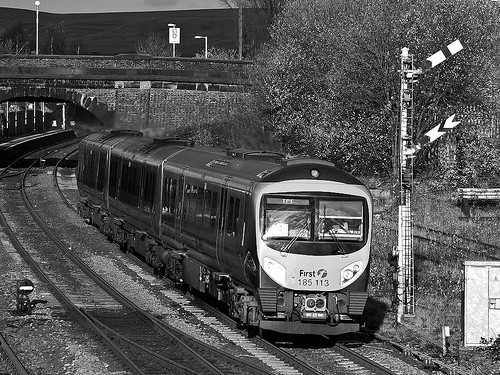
[75, 127, 374, 340]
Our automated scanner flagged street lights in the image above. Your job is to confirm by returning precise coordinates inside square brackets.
[34, 0, 40, 55]
[168, 23, 176, 58]
[194, 35, 208, 59]
